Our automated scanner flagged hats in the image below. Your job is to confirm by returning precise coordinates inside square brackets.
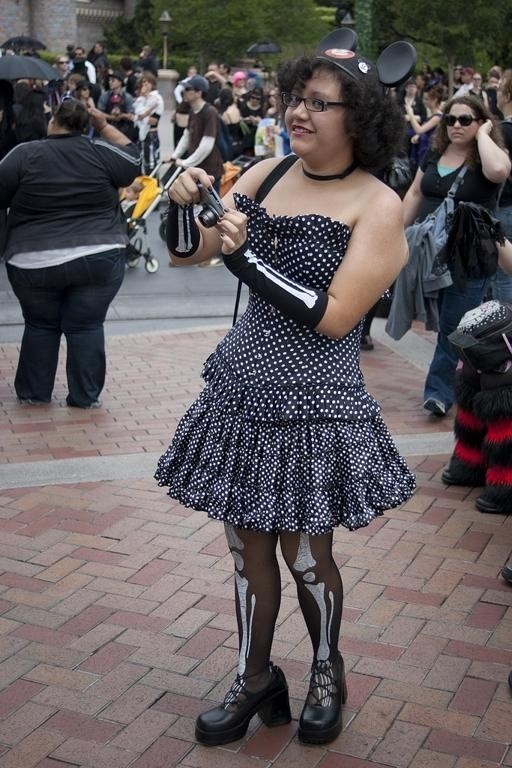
[107, 70, 127, 87]
[178, 75, 209, 93]
[312, 27, 418, 100]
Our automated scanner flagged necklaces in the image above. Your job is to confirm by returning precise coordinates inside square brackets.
[298, 161, 362, 181]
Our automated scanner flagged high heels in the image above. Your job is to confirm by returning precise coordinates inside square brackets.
[299, 651, 348, 745]
[194, 662, 292, 746]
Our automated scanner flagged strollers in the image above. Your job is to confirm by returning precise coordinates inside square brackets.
[120, 159, 182, 274]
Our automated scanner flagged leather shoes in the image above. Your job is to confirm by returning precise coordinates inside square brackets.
[475, 493, 508, 514]
[441, 469, 472, 486]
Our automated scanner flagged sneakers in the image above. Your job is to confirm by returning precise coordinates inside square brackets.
[423, 397, 446, 417]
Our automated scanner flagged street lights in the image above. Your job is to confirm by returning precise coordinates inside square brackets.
[158, 10, 173, 68]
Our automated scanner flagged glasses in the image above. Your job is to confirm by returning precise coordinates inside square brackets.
[281, 91, 349, 113]
[443, 113, 481, 127]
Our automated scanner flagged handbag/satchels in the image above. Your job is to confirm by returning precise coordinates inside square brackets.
[447, 298, 512, 374]
[383, 156, 413, 190]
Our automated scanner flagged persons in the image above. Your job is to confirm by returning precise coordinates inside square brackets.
[439, 199, 510, 279]
[392, 94, 512, 420]
[0, 22, 511, 354]
[154, 47, 419, 749]
[439, 299, 512, 514]
[0, 98, 141, 409]
[500, 561, 512, 690]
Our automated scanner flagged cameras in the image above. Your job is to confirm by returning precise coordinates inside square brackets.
[198, 182, 227, 229]
[479, 85, 497, 102]
